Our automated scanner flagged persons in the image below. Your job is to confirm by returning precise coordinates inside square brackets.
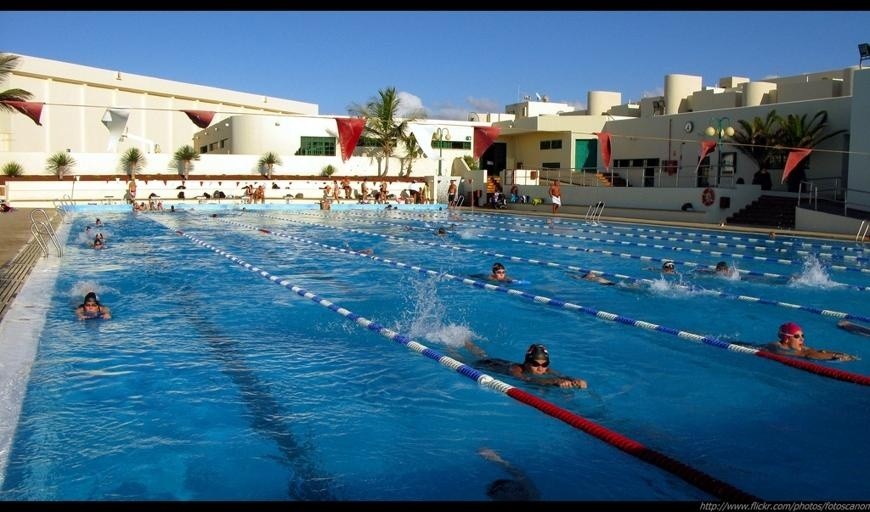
[470, 262, 521, 283]
[70, 291, 111, 322]
[315, 176, 458, 211]
[721, 323, 861, 363]
[485, 175, 518, 209]
[246, 184, 264, 200]
[769, 231, 777, 239]
[211, 214, 218, 217]
[566, 270, 637, 287]
[839, 320, 870, 336]
[693, 262, 745, 281]
[356, 249, 374, 254]
[639, 262, 678, 275]
[86, 218, 106, 250]
[475, 446, 541, 504]
[432, 228, 449, 238]
[130, 178, 177, 213]
[550, 179, 561, 214]
[445, 340, 589, 389]
[242, 208, 248, 212]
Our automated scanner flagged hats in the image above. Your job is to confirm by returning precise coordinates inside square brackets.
[493, 263, 504, 272]
[717, 262, 726, 270]
[526, 345, 549, 359]
[779, 323, 801, 338]
[664, 261, 674, 269]
[84, 292, 96, 302]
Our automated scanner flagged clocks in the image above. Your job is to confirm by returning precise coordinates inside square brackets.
[682, 120, 694, 134]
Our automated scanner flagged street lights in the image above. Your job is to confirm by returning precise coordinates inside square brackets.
[433, 127, 450, 183]
[705, 117, 736, 188]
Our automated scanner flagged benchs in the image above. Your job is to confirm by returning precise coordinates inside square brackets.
[9, 196, 419, 205]
[488, 200, 708, 223]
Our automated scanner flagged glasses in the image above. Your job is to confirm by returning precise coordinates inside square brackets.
[532, 361, 548, 366]
[781, 332, 804, 338]
